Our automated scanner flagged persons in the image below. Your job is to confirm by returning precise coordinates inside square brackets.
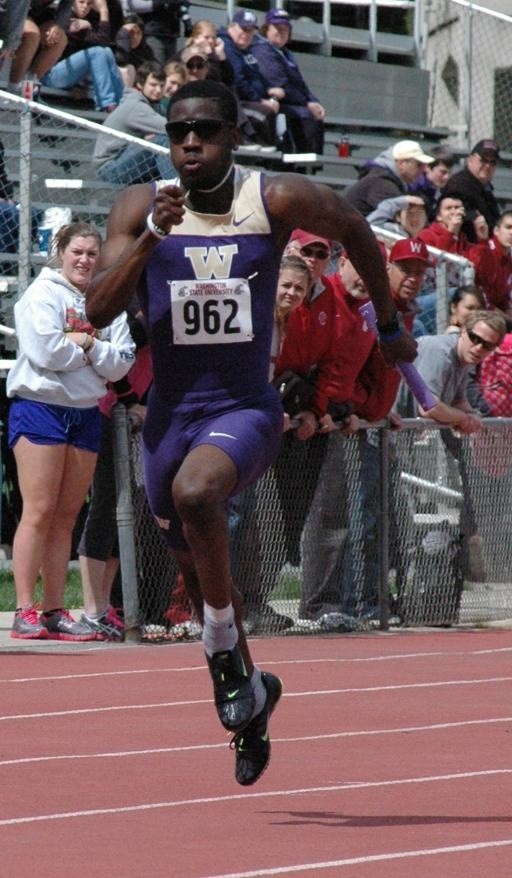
[338, 140, 503, 244]
[0, 187, 512, 641]
[0, 0, 327, 172]
[83, 80, 417, 785]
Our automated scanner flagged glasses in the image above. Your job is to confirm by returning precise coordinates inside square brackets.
[466, 329, 497, 351]
[290, 242, 331, 260]
[164, 118, 229, 143]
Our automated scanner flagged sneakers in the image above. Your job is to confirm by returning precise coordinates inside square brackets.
[204, 644, 256, 730]
[230, 674, 283, 785]
[14, 604, 291, 643]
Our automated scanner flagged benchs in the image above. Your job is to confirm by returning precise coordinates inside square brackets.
[0, 82, 511, 288]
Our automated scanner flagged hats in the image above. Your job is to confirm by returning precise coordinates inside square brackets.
[297, 234, 332, 247]
[390, 239, 430, 264]
[265, 8, 293, 24]
[179, 46, 209, 63]
[232, 9, 259, 28]
[470, 139, 502, 161]
[392, 140, 435, 164]
